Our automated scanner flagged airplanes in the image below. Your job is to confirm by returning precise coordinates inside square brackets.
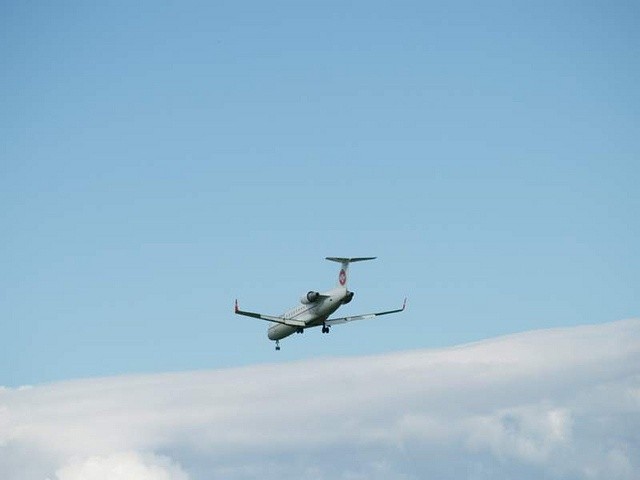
[235, 257, 407, 350]
